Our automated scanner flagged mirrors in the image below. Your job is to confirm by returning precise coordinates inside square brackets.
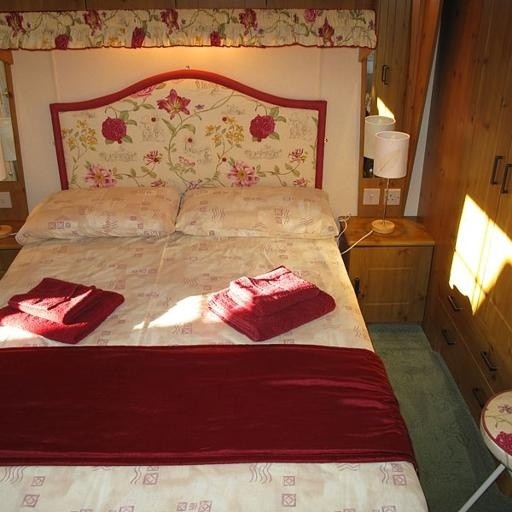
[0, 61, 20, 182]
[362, 0, 414, 180]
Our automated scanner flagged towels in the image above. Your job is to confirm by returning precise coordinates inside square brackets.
[8, 277, 103, 323]
[229, 265, 319, 316]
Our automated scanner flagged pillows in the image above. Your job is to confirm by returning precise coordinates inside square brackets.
[175, 185, 340, 240]
[15, 186, 181, 245]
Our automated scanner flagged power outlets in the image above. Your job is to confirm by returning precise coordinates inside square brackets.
[383, 188, 401, 206]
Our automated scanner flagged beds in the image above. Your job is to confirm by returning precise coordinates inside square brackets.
[0, 69, 430, 512]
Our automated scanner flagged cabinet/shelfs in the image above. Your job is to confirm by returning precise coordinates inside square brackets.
[417, 0, 512, 428]
[369, 0, 412, 133]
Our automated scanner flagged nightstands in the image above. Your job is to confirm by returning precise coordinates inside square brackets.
[0, 219, 26, 278]
[338, 215, 435, 324]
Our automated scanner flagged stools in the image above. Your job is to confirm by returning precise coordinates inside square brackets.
[457, 390, 512, 512]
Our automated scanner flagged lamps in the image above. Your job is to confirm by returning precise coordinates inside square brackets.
[364, 115, 397, 160]
[371, 131, 411, 234]
